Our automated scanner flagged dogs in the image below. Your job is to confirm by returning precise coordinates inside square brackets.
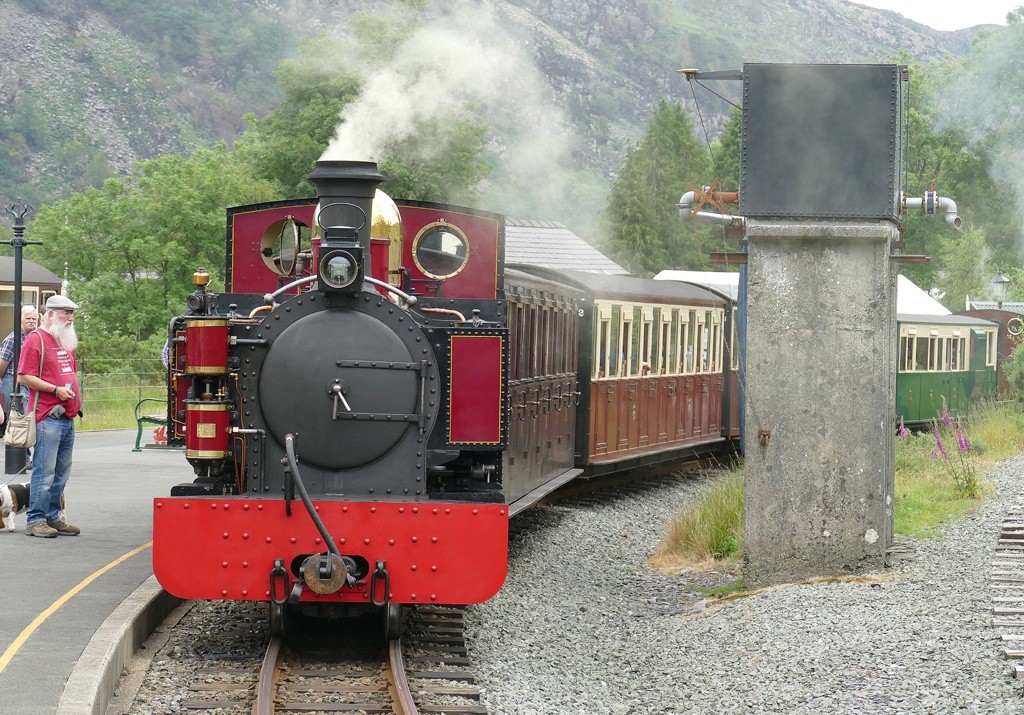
[0, 481, 65, 533]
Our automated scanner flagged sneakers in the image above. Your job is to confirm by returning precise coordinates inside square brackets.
[25, 521, 59, 537]
[50, 521, 81, 536]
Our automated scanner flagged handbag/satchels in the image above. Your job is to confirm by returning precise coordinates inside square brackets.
[3, 410, 37, 449]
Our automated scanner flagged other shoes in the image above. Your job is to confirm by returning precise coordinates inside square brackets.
[26, 457, 33, 469]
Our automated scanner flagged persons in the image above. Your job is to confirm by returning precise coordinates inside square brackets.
[0, 304, 40, 468]
[17, 294, 80, 537]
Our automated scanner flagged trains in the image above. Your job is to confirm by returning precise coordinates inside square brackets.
[149, 158, 1023, 642]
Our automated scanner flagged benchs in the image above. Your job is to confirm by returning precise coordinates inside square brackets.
[132, 398, 169, 451]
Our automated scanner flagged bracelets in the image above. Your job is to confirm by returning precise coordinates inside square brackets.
[53, 386, 57, 395]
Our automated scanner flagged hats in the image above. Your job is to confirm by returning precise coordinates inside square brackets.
[45, 295, 79, 310]
